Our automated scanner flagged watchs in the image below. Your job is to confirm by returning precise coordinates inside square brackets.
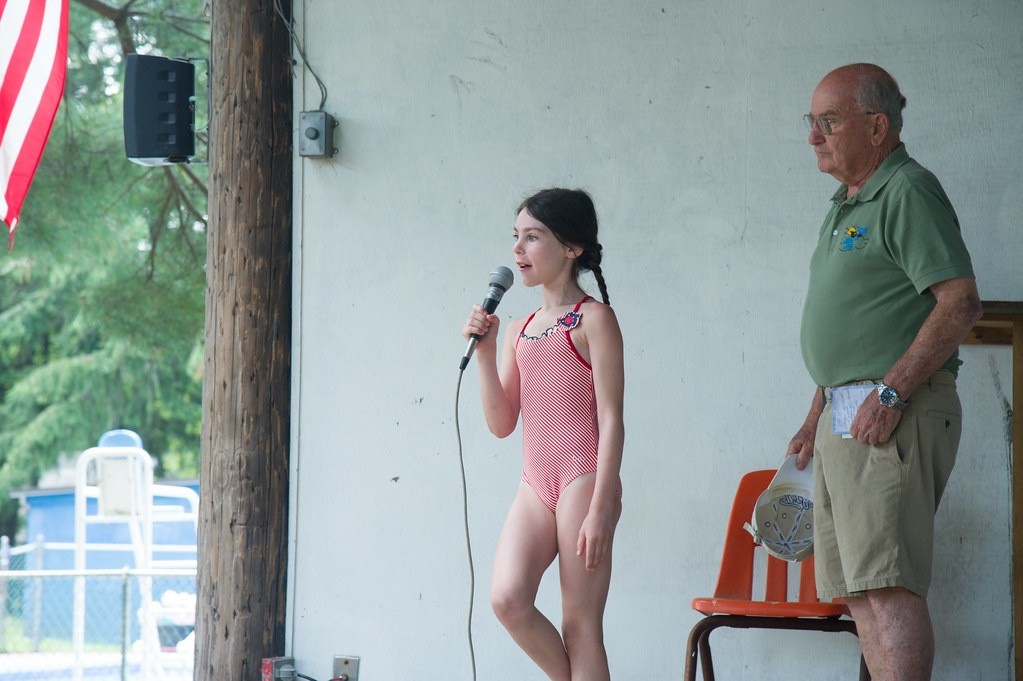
[877, 381, 909, 409]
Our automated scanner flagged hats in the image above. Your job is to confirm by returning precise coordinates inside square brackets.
[743, 454, 814, 564]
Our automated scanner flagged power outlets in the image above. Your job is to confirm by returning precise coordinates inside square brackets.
[262, 657, 295, 681]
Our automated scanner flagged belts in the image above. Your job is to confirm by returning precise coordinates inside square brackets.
[825, 370, 956, 403]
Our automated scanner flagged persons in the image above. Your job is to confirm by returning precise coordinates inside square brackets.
[461, 188, 624, 681]
[785, 63, 983, 681]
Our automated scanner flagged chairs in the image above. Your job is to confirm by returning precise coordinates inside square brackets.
[684, 469, 872, 681]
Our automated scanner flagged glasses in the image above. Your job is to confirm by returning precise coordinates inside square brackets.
[802, 110, 877, 135]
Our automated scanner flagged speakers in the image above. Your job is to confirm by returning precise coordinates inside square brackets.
[123, 53, 195, 166]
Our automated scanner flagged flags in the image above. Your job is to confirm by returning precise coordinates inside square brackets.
[0, 0, 71, 248]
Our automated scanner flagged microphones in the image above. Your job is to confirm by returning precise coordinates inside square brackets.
[460, 266, 514, 370]
